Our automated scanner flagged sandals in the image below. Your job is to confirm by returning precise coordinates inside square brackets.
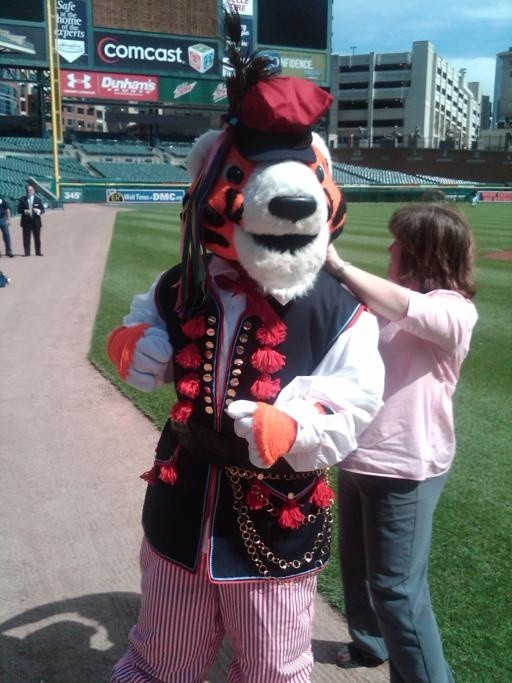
[336, 641, 364, 668]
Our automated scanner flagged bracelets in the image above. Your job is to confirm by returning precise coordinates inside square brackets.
[334, 261, 351, 278]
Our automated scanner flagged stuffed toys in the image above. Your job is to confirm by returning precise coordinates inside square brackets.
[110, 3, 387, 683]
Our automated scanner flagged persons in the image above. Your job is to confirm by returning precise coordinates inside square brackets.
[0, 195, 14, 256]
[324, 208, 478, 683]
[17, 186, 45, 256]
